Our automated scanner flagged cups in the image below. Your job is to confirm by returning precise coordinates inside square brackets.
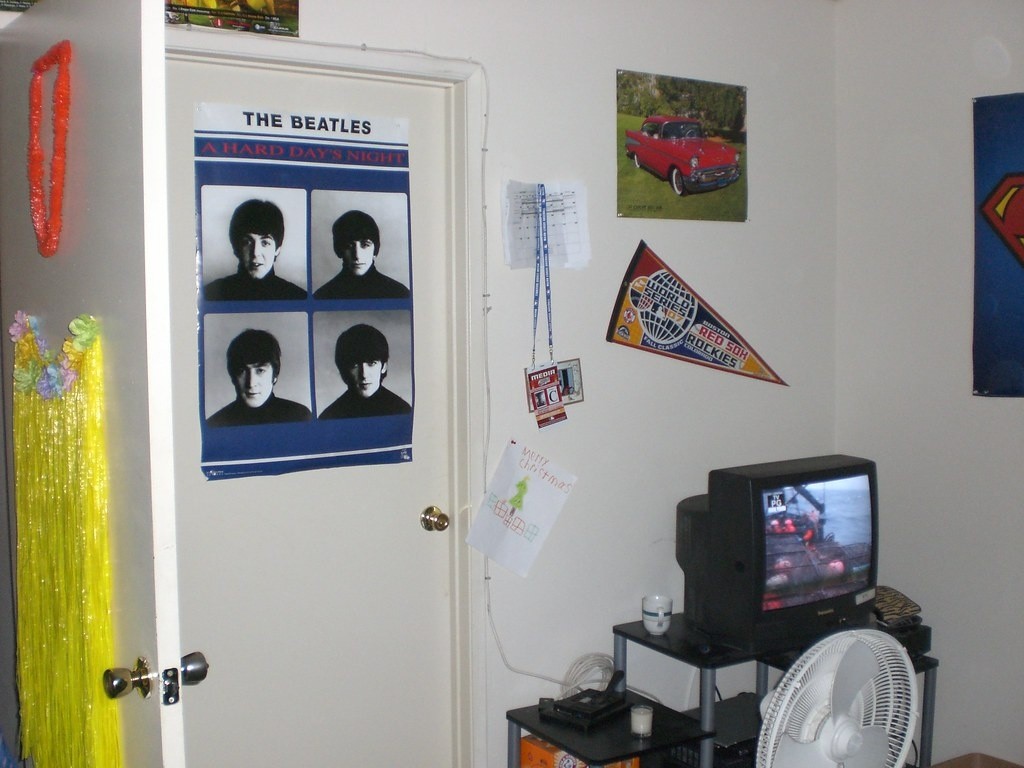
[630, 705, 653, 737]
[642, 595, 673, 635]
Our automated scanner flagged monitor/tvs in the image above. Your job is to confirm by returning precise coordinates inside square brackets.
[676, 455, 879, 654]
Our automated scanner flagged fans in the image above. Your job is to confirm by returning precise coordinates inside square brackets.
[755, 630, 918, 768]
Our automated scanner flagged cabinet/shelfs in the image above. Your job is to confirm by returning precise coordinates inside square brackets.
[506, 612, 939, 768]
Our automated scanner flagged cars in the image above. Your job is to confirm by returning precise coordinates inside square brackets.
[626, 114, 744, 197]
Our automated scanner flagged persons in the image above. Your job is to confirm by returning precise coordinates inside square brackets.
[200, 199, 310, 301]
[206, 328, 312, 428]
[316, 324, 412, 420]
[315, 210, 409, 300]
